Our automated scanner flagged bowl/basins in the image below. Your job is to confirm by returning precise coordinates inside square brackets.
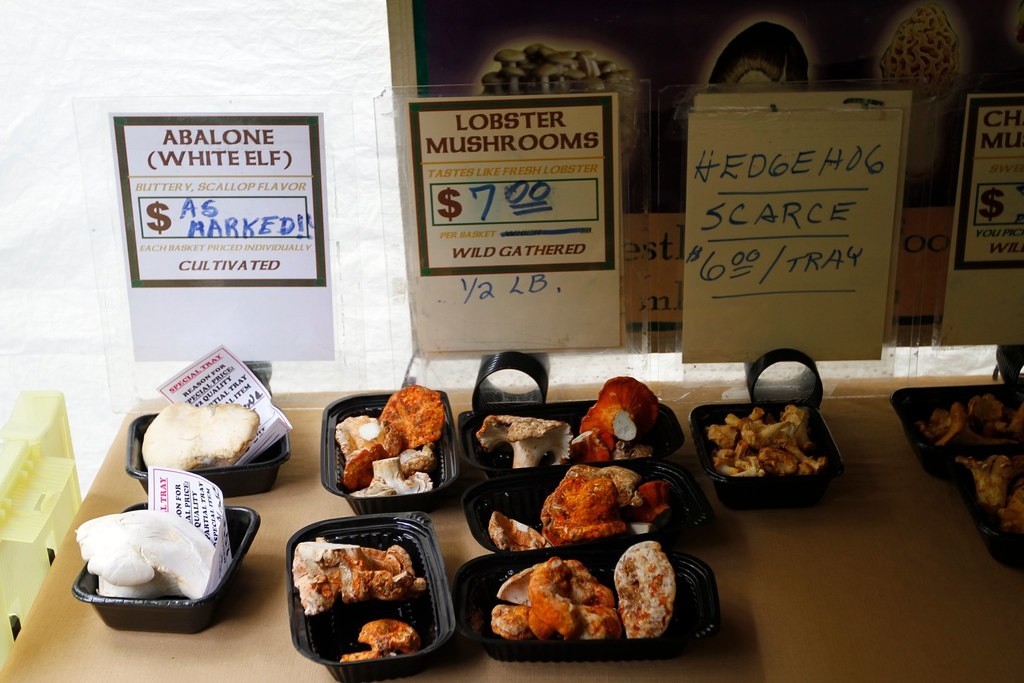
[320, 389, 458, 515]
[890, 384, 1023, 572]
[72, 499, 261, 634]
[689, 398, 844, 511]
[124, 413, 290, 497]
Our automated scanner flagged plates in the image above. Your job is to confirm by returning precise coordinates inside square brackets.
[282, 399, 721, 683]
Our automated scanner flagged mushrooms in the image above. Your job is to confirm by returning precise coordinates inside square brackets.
[918, 394, 1024, 535]
[76, 375, 827, 664]
